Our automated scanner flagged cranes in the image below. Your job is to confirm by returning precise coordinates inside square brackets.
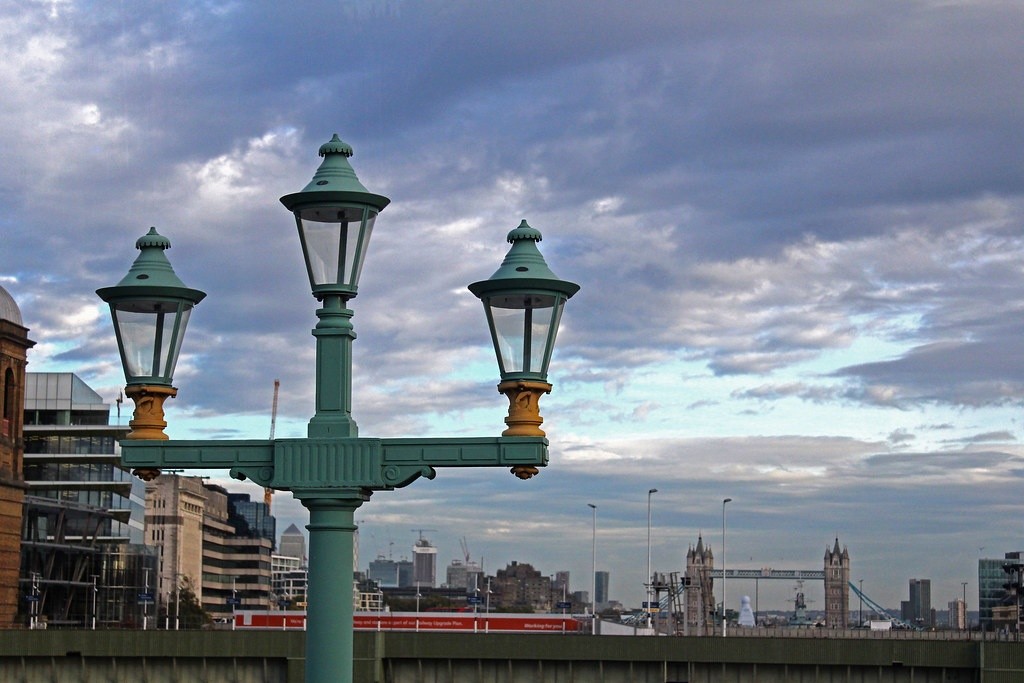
[263, 379, 280, 504]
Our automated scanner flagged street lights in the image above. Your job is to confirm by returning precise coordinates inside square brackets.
[646, 488, 658, 629]
[960, 581, 969, 631]
[94, 131, 580, 683]
[24, 560, 569, 636]
[721, 496, 732, 637]
[586, 503, 597, 635]
